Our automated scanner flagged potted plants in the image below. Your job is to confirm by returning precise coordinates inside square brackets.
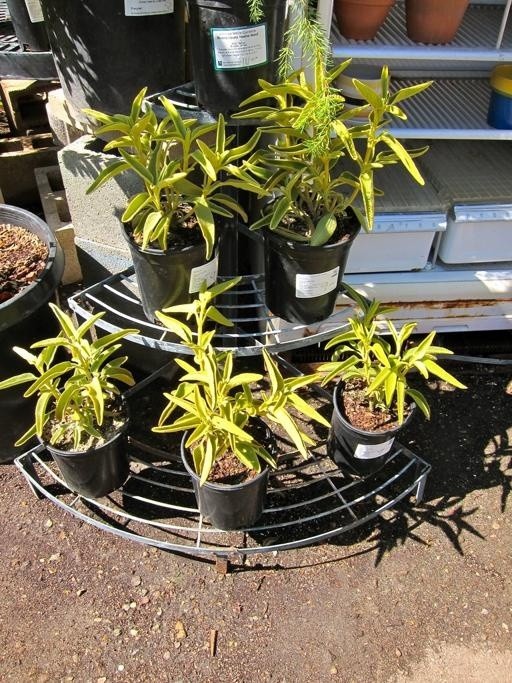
[228, 1, 437, 325]
[148, 274, 335, 532]
[2, 298, 143, 498]
[76, 83, 272, 326]
[307, 280, 473, 477]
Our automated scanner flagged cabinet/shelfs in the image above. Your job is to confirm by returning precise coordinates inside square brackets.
[253, 0, 511, 350]
[12, 78, 438, 576]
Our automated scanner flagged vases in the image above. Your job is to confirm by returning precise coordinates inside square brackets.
[483, 60, 511, 130]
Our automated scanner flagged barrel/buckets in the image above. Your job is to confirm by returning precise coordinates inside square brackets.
[264, 197, 359, 326]
[486, 64, 510, 128]
[180, 414, 273, 528]
[327, 376, 416, 476]
[188, 0, 287, 121]
[1, 203, 65, 463]
[119, 193, 228, 327]
[35, 384, 131, 496]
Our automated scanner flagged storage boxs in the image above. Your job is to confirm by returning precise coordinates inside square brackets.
[404, 137, 512, 267]
